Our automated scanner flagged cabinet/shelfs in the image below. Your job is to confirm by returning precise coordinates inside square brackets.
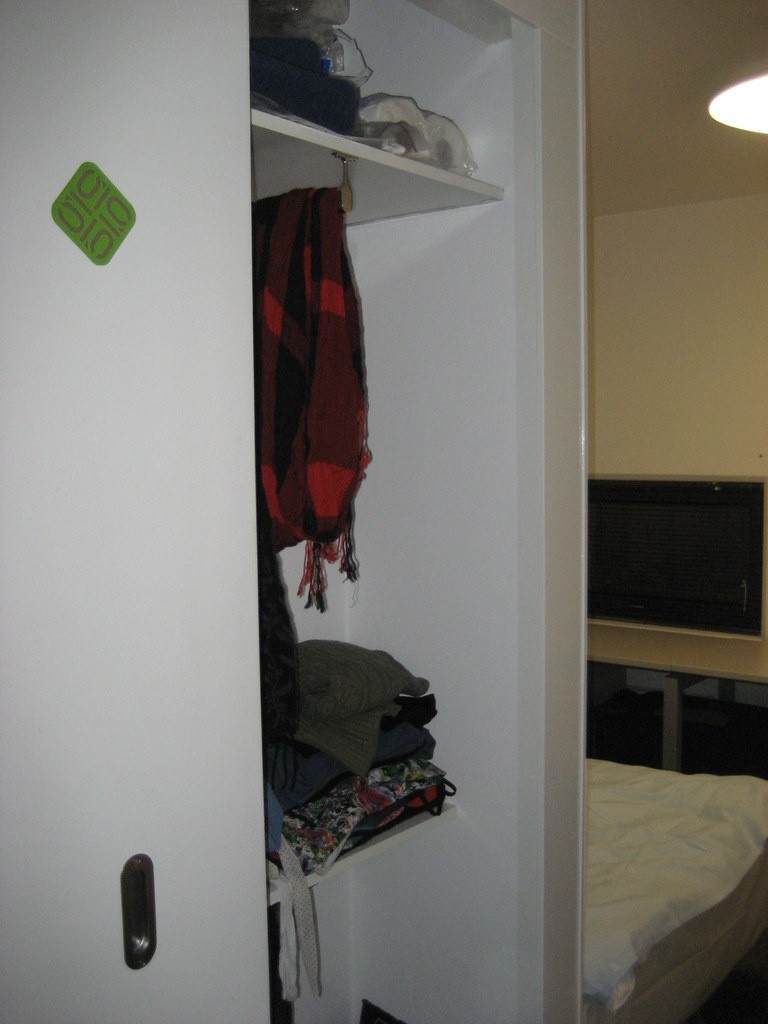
[0, 0, 594, 1024]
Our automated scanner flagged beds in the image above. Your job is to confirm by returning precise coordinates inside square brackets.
[585, 619, 767, 1023]
